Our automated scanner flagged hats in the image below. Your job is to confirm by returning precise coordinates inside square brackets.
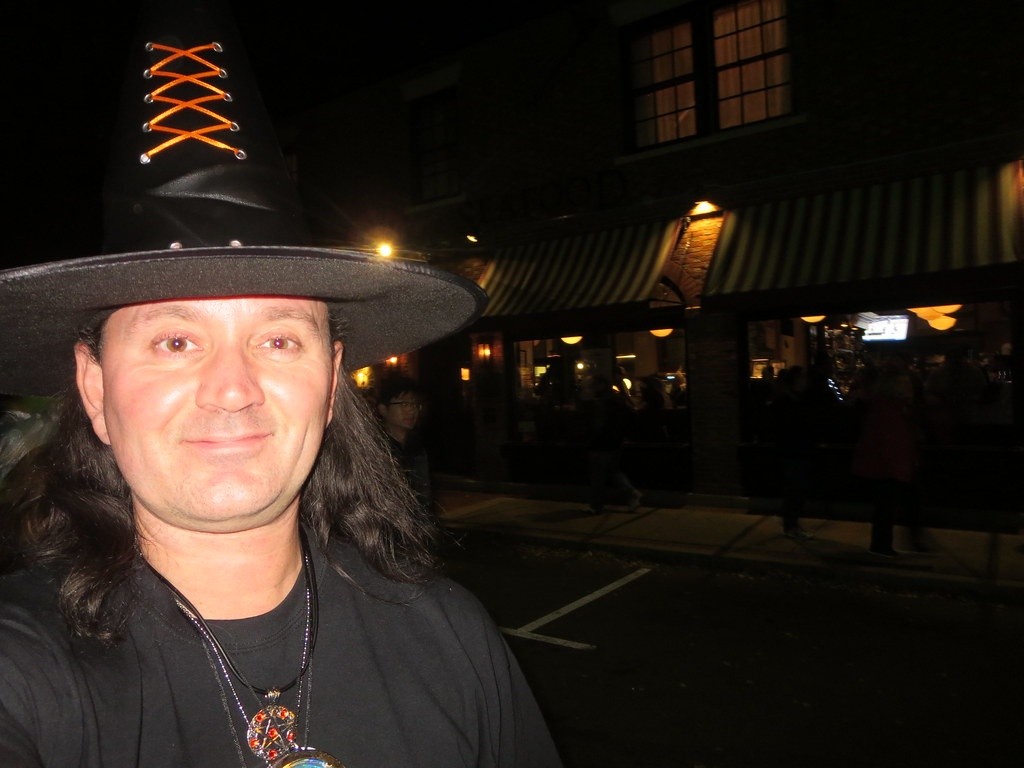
[0, 0, 490, 396]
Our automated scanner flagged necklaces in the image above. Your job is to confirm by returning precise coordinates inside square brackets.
[137, 524, 347, 768]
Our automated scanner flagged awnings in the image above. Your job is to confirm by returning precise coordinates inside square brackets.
[701, 156, 1023, 321]
[434, 216, 685, 317]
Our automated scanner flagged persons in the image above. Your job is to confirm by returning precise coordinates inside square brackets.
[766, 366, 815, 539]
[579, 379, 625, 515]
[0, 298, 568, 768]
[645, 361, 683, 408]
[863, 371, 934, 559]
[374, 376, 422, 492]
[797, 351, 973, 419]
